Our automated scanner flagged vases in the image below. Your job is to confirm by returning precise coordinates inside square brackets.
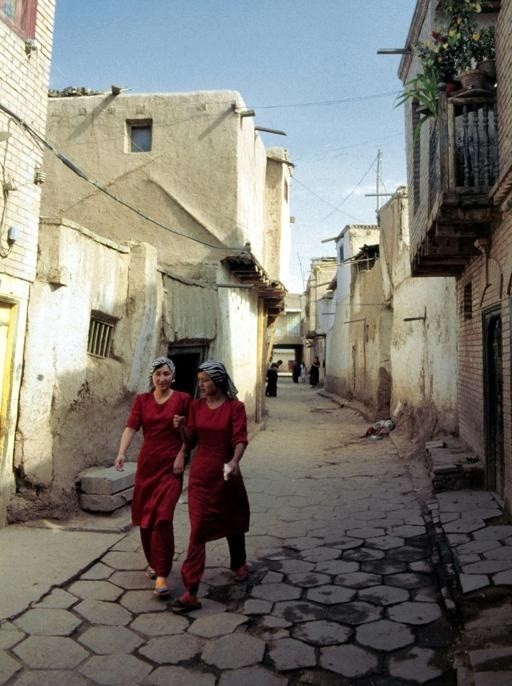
[444, 60, 492, 91]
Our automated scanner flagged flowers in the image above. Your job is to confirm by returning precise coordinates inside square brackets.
[417, 3, 500, 70]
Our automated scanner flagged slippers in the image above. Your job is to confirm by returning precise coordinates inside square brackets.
[166, 597, 201, 608]
[234, 564, 248, 581]
[152, 580, 170, 595]
[147, 565, 157, 579]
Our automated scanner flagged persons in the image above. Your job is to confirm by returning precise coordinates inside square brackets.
[171, 360, 252, 614]
[265, 356, 321, 398]
[112, 358, 194, 598]
